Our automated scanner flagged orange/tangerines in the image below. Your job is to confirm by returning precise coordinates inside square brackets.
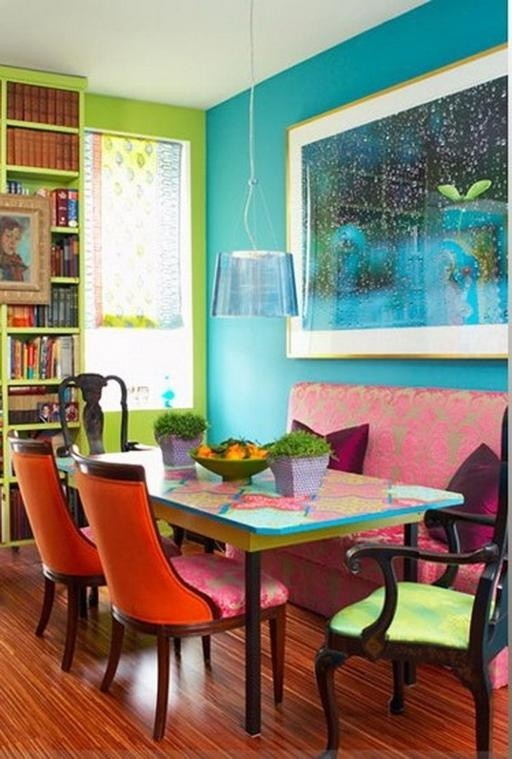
[196, 437, 276, 461]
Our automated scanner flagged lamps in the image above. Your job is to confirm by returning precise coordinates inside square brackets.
[211, 0, 301, 321]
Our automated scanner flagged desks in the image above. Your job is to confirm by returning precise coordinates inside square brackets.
[49, 450, 469, 735]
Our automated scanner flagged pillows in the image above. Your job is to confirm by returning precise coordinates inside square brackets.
[426, 440, 505, 551]
[289, 418, 370, 473]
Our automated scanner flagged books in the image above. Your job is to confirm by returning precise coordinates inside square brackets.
[8, 386, 78, 425]
[36, 187, 78, 227]
[8, 335, 80, 379]
[7, 81, 79, 127]
[62, 485, 90, 528]
[9, 488, 33, 541]
[52, 235, 79, 276]
[7, 284, 79, 327]
[7, 127, 79, 171]
[7, 181, 29, 196]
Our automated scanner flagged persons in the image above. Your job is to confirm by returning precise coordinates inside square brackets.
[0, 216, 27, 281]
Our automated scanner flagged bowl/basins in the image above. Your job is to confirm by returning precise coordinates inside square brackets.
[190, 444, 276, 488]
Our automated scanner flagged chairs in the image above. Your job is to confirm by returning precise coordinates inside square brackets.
[54, 372, 141, 608]
[313, 402, 511, 758]
[67, 443, 292, 743]
[4, 427, 182, 671]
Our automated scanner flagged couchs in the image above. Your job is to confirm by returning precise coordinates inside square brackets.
[223, 383, 512, 691]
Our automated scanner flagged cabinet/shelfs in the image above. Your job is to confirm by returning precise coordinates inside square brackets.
[0, 64, 89, 549]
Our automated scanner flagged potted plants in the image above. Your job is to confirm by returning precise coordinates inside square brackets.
[154, 410, 213, 470]
[258, 432, 334, 498]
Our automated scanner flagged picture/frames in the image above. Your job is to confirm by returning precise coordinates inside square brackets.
[1, 193, 54, 305]
[286, 44, 510, 362]
